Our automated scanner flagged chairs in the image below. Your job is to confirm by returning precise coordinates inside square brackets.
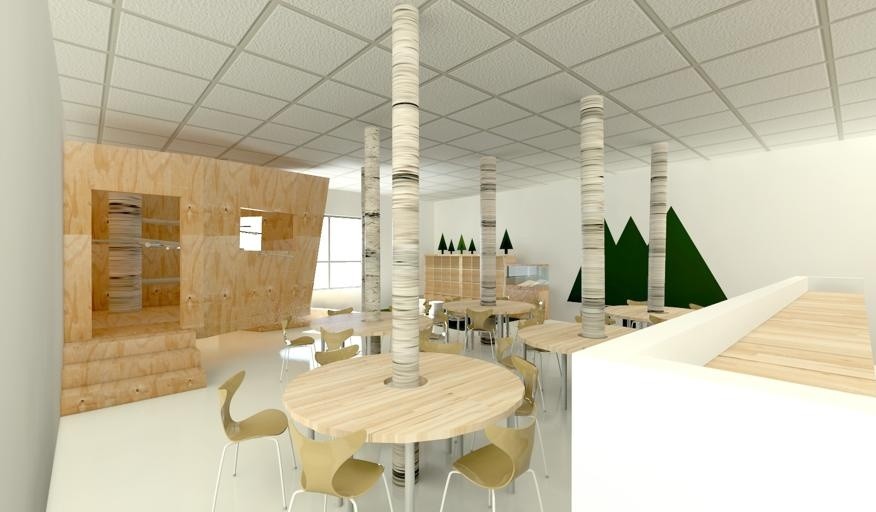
[212, 370, 297, 512]
[314, 344, 359, 367]
[287, 420, 393, 512]
[648, 315, 666, 324]
[689, 304, 701, 310]
[521, 344, 562, 392]
[279, 315, 319, 381]
[320, 326, 353, 352]
[627, 299, 648, 327]
[511, 307, 544, 355]
[465, 307, 496, 361]
[328, 307, 353, 316]
[419, 340, 464, 355]
[439, 416, 548, 512]
[508, 355, 546, 416]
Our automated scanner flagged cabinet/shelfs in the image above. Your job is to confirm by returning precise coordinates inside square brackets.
[424, 253, 517, 301]
